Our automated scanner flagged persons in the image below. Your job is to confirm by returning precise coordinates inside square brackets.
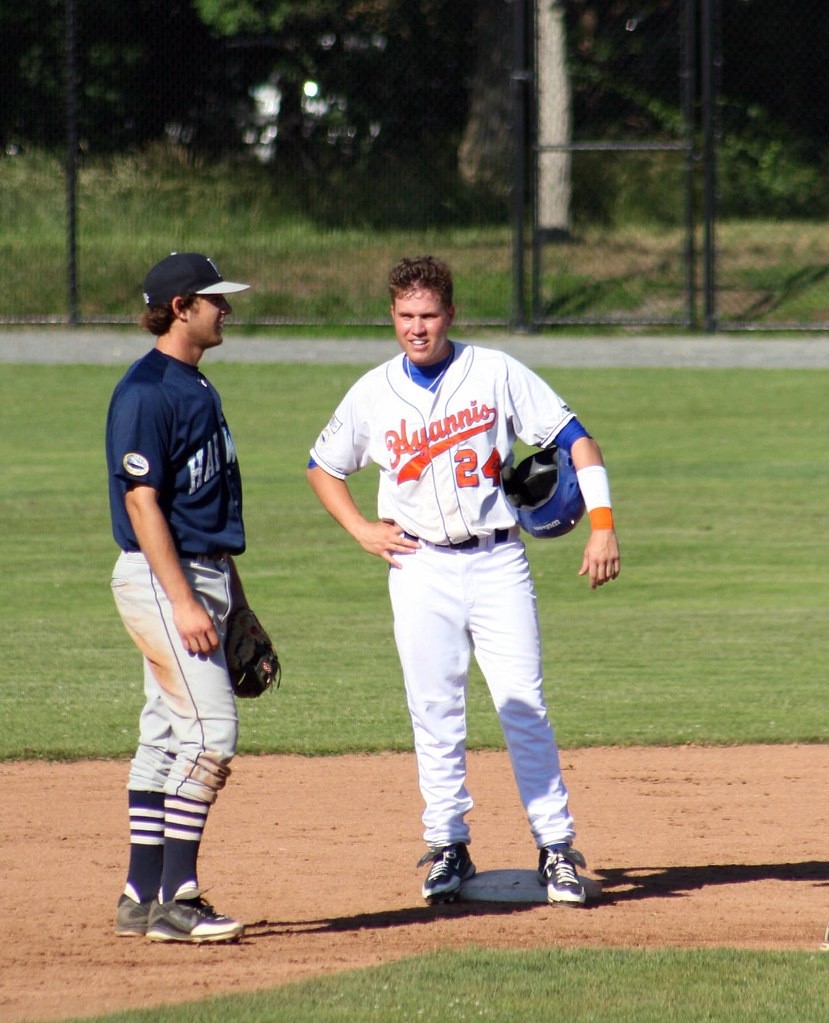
[100, 253, 253, 943]
[303, 257, 617, 904]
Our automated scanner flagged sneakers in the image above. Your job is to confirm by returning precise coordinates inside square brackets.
[422, 841, 476, 905]
[144, 884, 245, 946]
[114, 894, 151, 937]
[537, 845, 586, 908]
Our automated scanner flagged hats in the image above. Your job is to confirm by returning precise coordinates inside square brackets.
[143, 252, 252, 311]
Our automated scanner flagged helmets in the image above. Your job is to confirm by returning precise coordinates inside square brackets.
[498, 443, 585, 540]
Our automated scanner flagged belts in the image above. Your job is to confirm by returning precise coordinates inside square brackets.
[403, 528, 508, 551]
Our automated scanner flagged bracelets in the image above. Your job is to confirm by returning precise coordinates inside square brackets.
[588, 507, 614, 530]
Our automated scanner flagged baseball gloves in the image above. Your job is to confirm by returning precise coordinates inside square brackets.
[219, 566, 279, 700]
